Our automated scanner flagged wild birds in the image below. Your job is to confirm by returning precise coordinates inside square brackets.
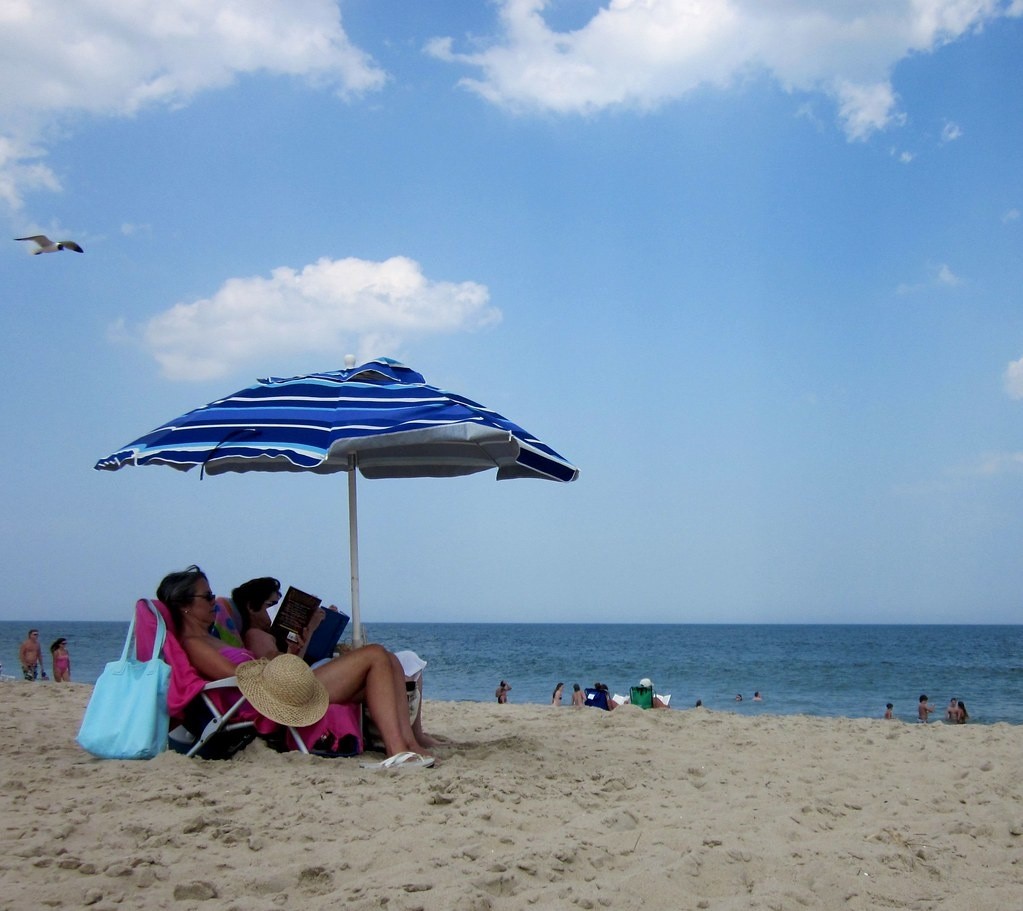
[15, 234, 84, 256]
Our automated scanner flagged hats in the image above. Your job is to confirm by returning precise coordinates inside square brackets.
[236, 659, 330, 727]
[640, 678, 655, 687]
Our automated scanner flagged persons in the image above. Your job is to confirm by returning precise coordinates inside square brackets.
[552, 682, 564, 706]
[918, 695, 935, 724]
[884, 703, 893, 719]
[156, 564, 438, 770]
[50, 638, 71, 682]
[228, 577, 451, 748]
[495, 680, 512, 703]
[19, 629, 44, 681]
[572, 685, 587, 706]
[944, 698, 970, 724]
[597, 679, 762, 711]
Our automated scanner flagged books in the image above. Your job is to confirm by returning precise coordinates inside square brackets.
[613, 694, 631, 705]
[269, 585, 350, 666]
[653, 693, 671, 706]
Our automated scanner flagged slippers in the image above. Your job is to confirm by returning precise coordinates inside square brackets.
[358, 754, 434, 772]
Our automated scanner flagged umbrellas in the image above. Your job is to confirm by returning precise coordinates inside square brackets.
[93, 355, 580, 734]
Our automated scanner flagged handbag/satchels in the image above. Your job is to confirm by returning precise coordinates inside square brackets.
[74, 595, 170, 760]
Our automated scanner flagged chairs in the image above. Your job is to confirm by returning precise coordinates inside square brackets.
[584, 688, 612, 712]
[135, 597, 364, 759]
[630, 686, 653, 709]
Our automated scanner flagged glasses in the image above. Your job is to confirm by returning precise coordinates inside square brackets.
[30, 633, 39, 636]
[59, 642, 67, 646]
[262, 600, 277, 607]
[182, 593, 215, 603]
